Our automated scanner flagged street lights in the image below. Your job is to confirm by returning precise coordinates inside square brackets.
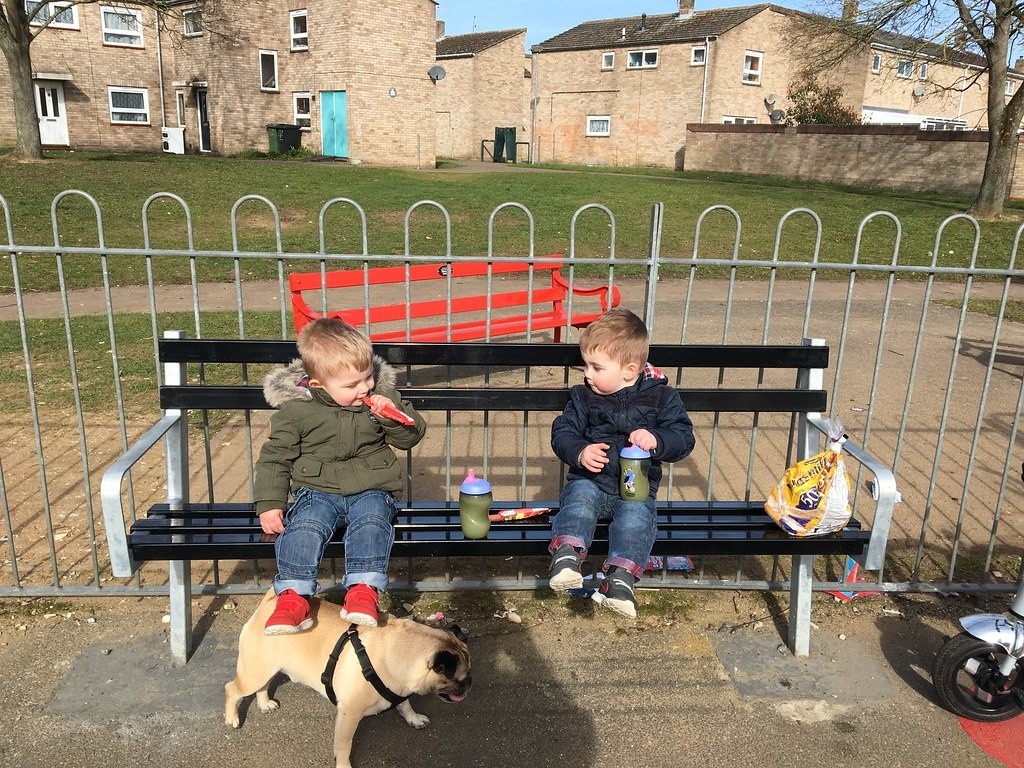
[531, 46, 543, 165]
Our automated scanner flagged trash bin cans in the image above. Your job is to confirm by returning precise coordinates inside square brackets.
[267, 123, 302, 155]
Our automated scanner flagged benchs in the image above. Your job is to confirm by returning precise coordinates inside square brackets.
[289, 255, 621, 344]
[100, 329, 896, 666]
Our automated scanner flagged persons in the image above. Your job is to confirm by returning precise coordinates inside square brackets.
[549, 308, 696, 618]
[253, 318, 426, 635]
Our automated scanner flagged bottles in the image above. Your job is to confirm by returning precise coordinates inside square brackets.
[619, 443, 652, 501]
[459, 469, 493, 539]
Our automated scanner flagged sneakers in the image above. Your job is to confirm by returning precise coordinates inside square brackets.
[590, 568, 637, 619]
[340, 583, 378, 628]
[548, 543, 584, 592]
[264, 589, 313, 636]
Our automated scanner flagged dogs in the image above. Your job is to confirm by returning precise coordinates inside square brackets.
[223, 585, 473, 768]
